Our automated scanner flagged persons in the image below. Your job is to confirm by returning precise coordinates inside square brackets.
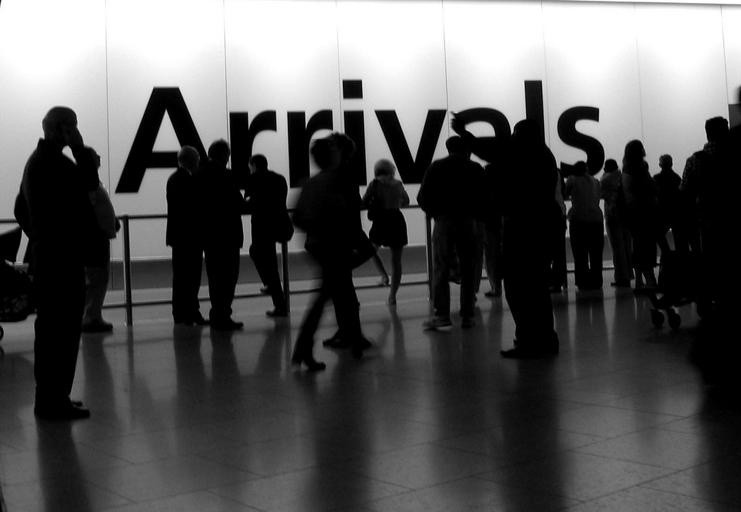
[66, 146, 121, 335]
[14, 104, 119, 425]
[165, 113, 741, 374]
